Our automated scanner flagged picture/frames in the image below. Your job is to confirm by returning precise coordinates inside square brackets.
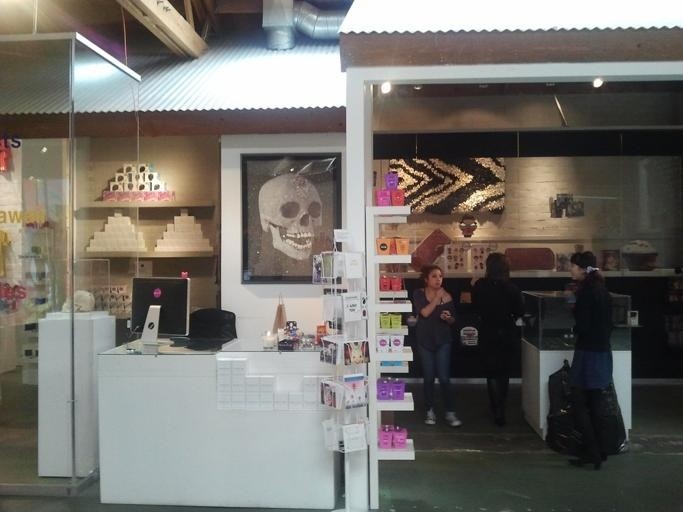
[239, 151, 344, 286]
[601, 250, 620, 271]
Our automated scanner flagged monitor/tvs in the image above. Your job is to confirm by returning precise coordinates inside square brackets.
[131, 277, 190, 346]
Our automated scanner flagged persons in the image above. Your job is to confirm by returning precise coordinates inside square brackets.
[467, 252, 527, 428]
[565, 250, 615, 471]
[406, 262, 463, 428]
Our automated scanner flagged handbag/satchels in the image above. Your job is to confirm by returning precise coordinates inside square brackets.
[171, 307, 237, 354]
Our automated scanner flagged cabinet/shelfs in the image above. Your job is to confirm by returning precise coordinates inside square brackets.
[365, 204, 415, 512]
[21, 199, 220, 386]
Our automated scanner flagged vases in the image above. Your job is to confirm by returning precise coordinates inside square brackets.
[622, 252, 658, 271]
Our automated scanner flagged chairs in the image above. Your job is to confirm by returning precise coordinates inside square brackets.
[189, 308, 237, 339]
[504, 248, 555, 270]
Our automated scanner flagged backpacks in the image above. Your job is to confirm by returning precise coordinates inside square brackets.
[544, 360, 605, 463]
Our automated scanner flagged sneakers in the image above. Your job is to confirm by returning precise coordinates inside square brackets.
[423, 408, 437, 425]
[441, 410, 463, 428]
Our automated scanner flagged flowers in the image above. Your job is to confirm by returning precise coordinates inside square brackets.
[623, 240, 657, 253]
